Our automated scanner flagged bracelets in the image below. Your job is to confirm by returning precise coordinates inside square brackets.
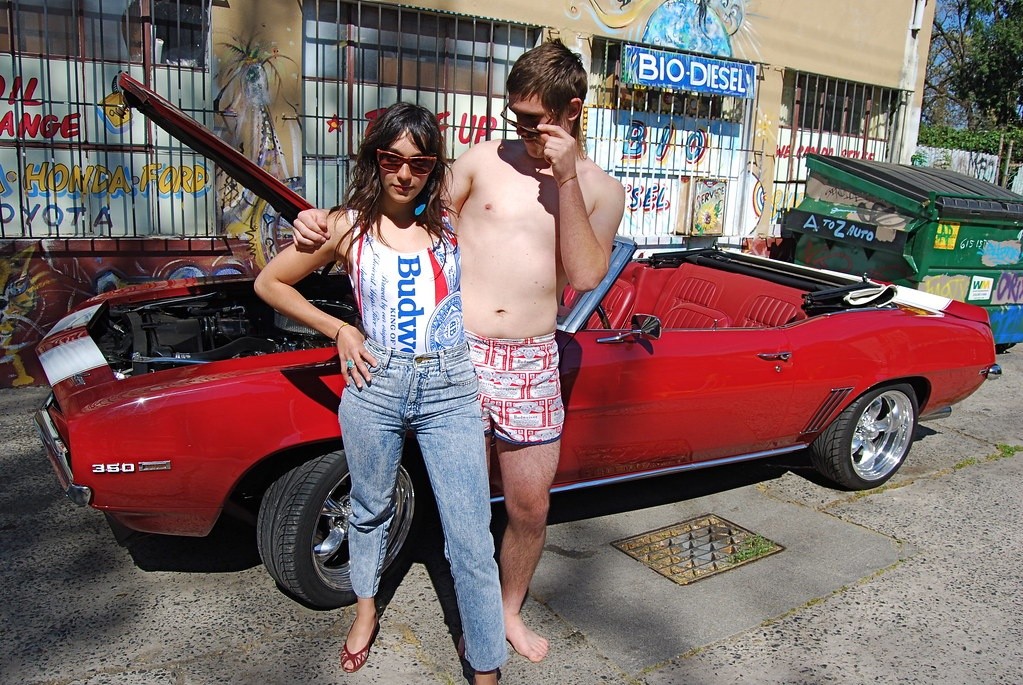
[560, 174, 576, 188]
[335, 322, 348, 340]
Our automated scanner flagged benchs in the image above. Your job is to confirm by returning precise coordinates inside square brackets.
[651, 262, 814, 327]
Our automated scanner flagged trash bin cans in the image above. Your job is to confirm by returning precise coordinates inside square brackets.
[779, 152, 1023, 356]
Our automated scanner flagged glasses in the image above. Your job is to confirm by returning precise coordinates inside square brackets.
[377, 148, 437, 176]
[500, 102, 557, 135]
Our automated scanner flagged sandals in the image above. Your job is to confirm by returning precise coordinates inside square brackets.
[340, 614, 378, 672]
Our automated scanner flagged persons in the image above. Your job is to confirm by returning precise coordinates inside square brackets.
[254, 101, 507, 685]
[292, 38, 625, 663]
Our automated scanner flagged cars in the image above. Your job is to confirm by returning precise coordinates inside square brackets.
[32, 235, 1004, 609]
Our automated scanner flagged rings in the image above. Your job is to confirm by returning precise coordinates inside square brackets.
[346, 360, 354, 369]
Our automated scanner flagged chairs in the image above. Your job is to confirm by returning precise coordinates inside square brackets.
[661, 302, 732, 330]
[563, 278, 636, 329]
